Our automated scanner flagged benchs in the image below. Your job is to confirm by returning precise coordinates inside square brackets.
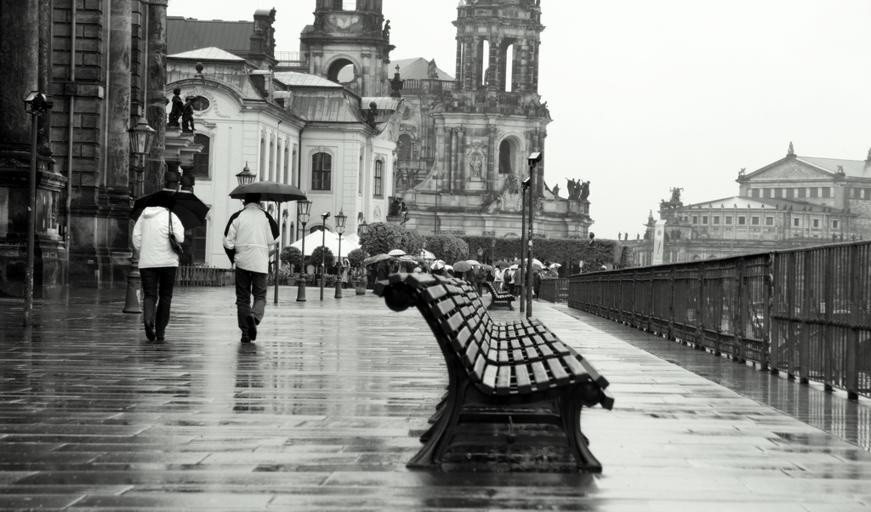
[373, 271, 613, 473]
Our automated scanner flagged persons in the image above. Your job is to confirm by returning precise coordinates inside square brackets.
[223, 197, 280, 341]
[168, 88, 184, 124]
[182, 96, 197, 132]
[336, 247, 562, 300]
[130, 192, 185, 342]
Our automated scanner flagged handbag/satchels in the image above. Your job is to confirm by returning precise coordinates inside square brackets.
[168, 233, 183, 254]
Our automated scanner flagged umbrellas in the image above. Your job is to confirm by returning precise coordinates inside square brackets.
[229, 181, 308, 202]
[132, 189, 210, 231]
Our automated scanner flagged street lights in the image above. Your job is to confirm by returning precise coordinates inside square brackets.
[521, 151, 542, 319]
[123, 112, 158, 314]
[358, 220, 370, 294]
[320, 211, 330, 301]
[335, 207, 347, 297]
[477, 239, 496, 297]
[235, 161, 257, 205]
[296, 193, 313, 301]
[21, 90, 47, 343]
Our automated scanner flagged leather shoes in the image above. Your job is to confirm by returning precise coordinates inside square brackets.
[145, 321, 155, 341]
[157, 326, 164, 341]
[238, 314, 257, 343]
